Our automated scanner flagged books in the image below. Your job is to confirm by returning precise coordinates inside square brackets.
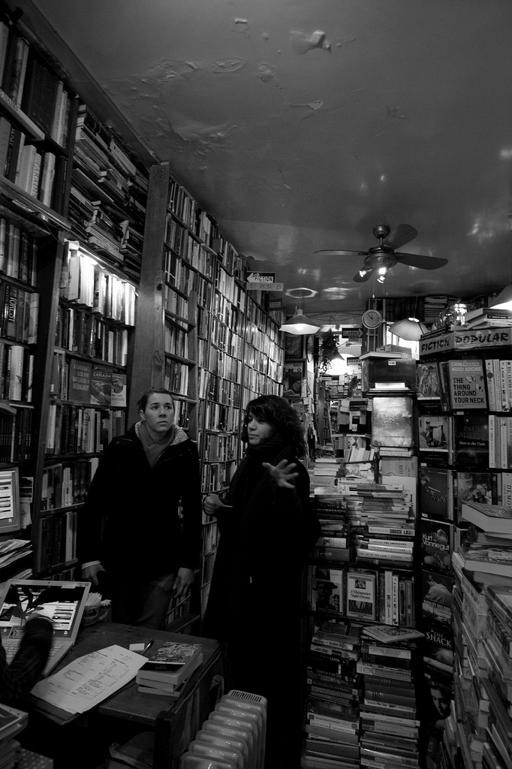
[301, 446, 453, 768]
[51, 238, 139, 406]
[439, 501, 512, 769]
[165, 185, 215, 428]
[135, 640, 203, 698]
[413, 354, 512, 501]
[40, 410, 128, 568]
[0, 7, 151, 269]
[246, 286, 286, 399]
[0, 208, 50, 585]
[200, 209, 248, 493]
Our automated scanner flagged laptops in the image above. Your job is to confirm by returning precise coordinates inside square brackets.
[0, 579, 91, 674]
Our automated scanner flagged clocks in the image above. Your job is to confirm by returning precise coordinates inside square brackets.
[361, 309, 383, 330]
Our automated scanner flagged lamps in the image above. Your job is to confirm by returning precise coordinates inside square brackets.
[326, 351, 347, 376]
[388, 316, 426, 342]
[278, 287, 321, 335]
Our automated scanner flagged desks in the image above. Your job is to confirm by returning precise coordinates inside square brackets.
[28, 615, 223, 761]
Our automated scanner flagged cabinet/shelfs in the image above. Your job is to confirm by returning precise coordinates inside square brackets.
[417, 296, 486, 327]
[162, 173, 286, 616]
[1, 0, 171, 616]
[407, 315, 512, 769]
[308, 314, 372, 459]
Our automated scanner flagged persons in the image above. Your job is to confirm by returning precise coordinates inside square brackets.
[202, 395, 321, 769]
[0, 612, 54, 710]
[77, 388, 201, 627]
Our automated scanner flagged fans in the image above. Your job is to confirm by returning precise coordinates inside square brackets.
[313, 224, 448, 282]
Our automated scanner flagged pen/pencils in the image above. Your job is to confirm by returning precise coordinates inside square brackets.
[137, 639, 155, 656]
